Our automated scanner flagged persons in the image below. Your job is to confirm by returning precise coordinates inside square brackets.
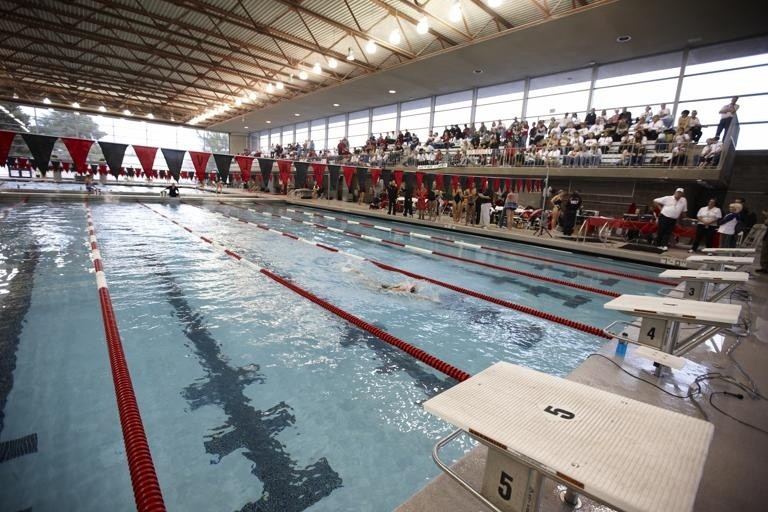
[351, 268, 441, 304]
[336, 116, 529, 230]
[756, 208, 768, 274]
[162, 183, 180, 198]
[85, 175, 97, 191]
[211, 140, 328, 200]
[528, 97, 747, 257]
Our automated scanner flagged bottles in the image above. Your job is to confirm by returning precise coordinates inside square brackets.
[614, 333, 628, 360]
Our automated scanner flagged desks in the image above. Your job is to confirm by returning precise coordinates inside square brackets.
[587, 216, 721, 252]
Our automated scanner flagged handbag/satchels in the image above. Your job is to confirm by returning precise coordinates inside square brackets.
[735, 223, 742, 233]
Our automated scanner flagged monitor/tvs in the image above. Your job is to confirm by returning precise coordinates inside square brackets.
[641, 214, 654, 221]
[624, 214, 639, 221]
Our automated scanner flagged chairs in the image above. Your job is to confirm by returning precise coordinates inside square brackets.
[6, 155, 32, 177]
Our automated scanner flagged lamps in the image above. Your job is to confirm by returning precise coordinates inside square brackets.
[346, 29, 355, 60]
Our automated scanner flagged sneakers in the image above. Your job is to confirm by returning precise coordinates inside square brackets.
[658, 247, 668, 251]
[755, 269, 767, 273]
[688, 249, 693, 253]
[708, 252, 713, 256]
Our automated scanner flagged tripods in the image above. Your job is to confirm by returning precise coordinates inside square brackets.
[533, 179, 552, 238]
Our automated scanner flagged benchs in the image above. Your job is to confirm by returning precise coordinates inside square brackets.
[435, 137, 492, 166]
[599, 121, 674, 166]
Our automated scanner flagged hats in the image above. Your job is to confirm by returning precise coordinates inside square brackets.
[676, 188, 684, 192]
